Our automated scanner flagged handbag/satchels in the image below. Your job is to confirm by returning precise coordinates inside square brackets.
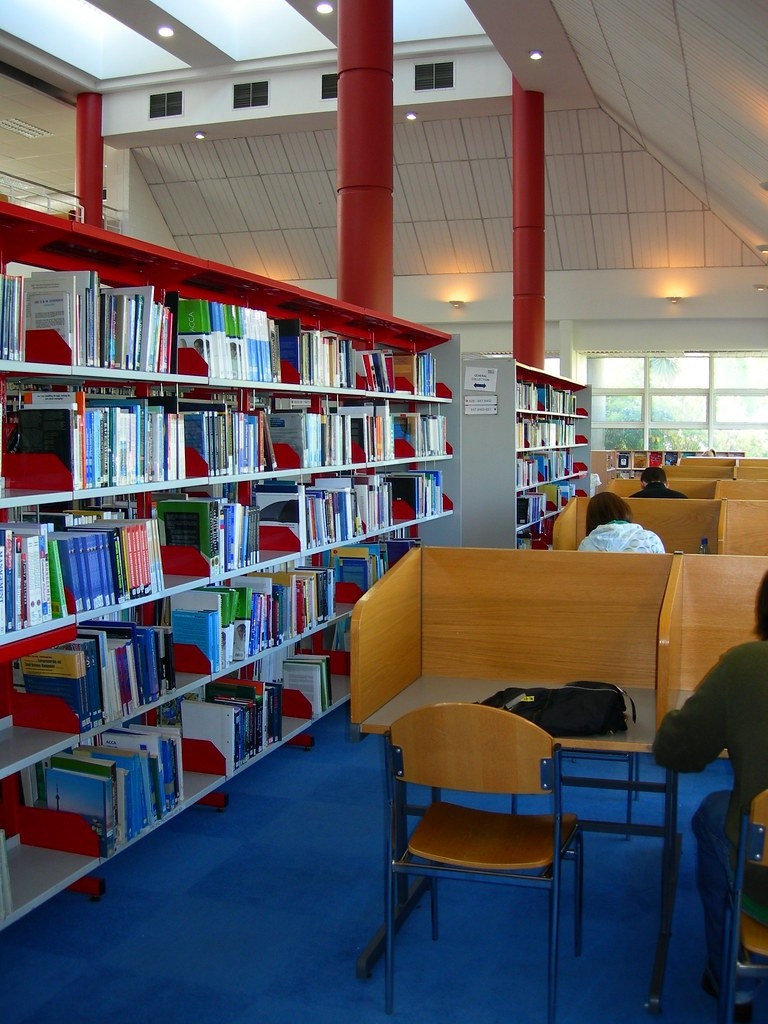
[473, 680, 636, 735]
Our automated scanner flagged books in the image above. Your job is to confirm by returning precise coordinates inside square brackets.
[617, 452, 678, 479]
[0, 263, 577, 852]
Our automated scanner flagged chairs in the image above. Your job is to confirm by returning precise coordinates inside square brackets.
[705, 792, 768, 1024]
[381, 702, 584, 1024]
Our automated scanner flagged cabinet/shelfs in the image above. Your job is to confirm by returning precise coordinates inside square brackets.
[0, 197, 453, 935]
[591, 449, 745, 498]
[514, 359, 591, 551]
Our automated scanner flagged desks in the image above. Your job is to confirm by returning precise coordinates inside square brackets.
[348, 458, 768, 1014]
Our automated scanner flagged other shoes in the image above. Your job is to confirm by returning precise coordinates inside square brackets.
[702, 975, 755, 1024]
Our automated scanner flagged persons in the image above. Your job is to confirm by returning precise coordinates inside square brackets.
[629, 466, 688, 498]
[702, 448, 715, 457]
[652, 564, 768, 1024]
[576, 492, 666, 555]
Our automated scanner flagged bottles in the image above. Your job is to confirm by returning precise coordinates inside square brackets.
[697, 538, 711, 554]
